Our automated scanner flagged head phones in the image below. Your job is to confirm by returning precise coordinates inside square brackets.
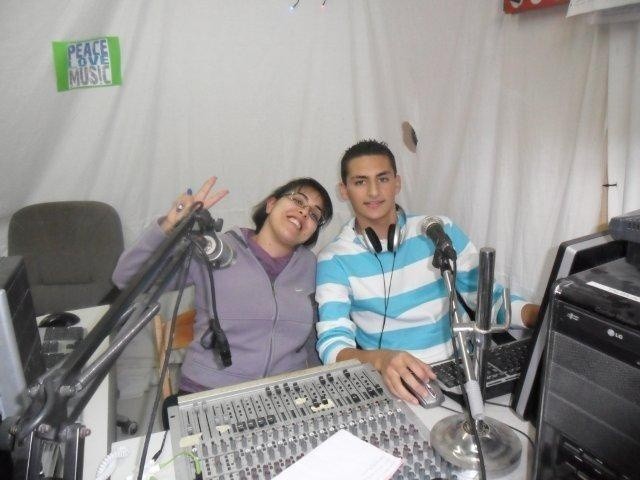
[354, 202, 409, 254]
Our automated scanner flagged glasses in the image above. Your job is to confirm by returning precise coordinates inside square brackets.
[280, 192, 326, 226]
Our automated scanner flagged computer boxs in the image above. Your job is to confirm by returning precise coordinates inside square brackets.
[0, 254, 43, 419]
[532, 256, 640, 480]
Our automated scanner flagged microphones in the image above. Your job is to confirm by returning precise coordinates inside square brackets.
[197, 207, 238, 269]
[419, 213, 457, 262]
[214, 322, 233, 369]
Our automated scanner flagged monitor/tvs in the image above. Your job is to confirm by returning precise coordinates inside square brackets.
[508, 229, 625, 422]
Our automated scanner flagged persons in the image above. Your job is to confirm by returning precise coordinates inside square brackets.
[312, 136, 542, 407]
[109, 176, 334, 429]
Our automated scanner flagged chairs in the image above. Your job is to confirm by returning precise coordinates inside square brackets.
[6, 199, 127, 319]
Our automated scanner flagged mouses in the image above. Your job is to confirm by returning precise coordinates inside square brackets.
[37, 311, 80, 326]
[402, 371, 446, 409]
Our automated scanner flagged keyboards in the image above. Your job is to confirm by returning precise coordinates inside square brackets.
[39, 326, 89, 375]
[430, 335, 530, 405]
